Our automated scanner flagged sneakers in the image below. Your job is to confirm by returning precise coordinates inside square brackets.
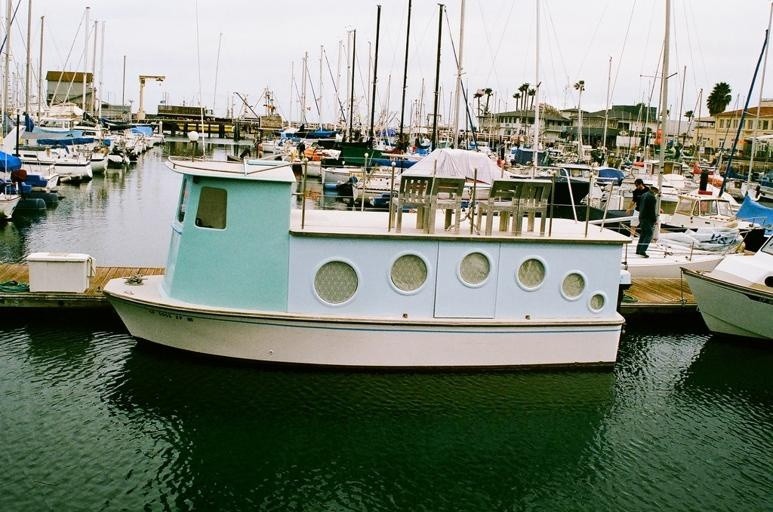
[636, 252, 649, 258]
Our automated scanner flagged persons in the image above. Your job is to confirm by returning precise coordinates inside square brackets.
[625, 176, 663, 240]
[636, 183, 660, 258]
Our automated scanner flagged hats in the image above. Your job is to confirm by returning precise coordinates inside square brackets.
[651, 184, 659, 190]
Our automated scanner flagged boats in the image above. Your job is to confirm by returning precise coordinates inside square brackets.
[101, 149, 629, 374]
